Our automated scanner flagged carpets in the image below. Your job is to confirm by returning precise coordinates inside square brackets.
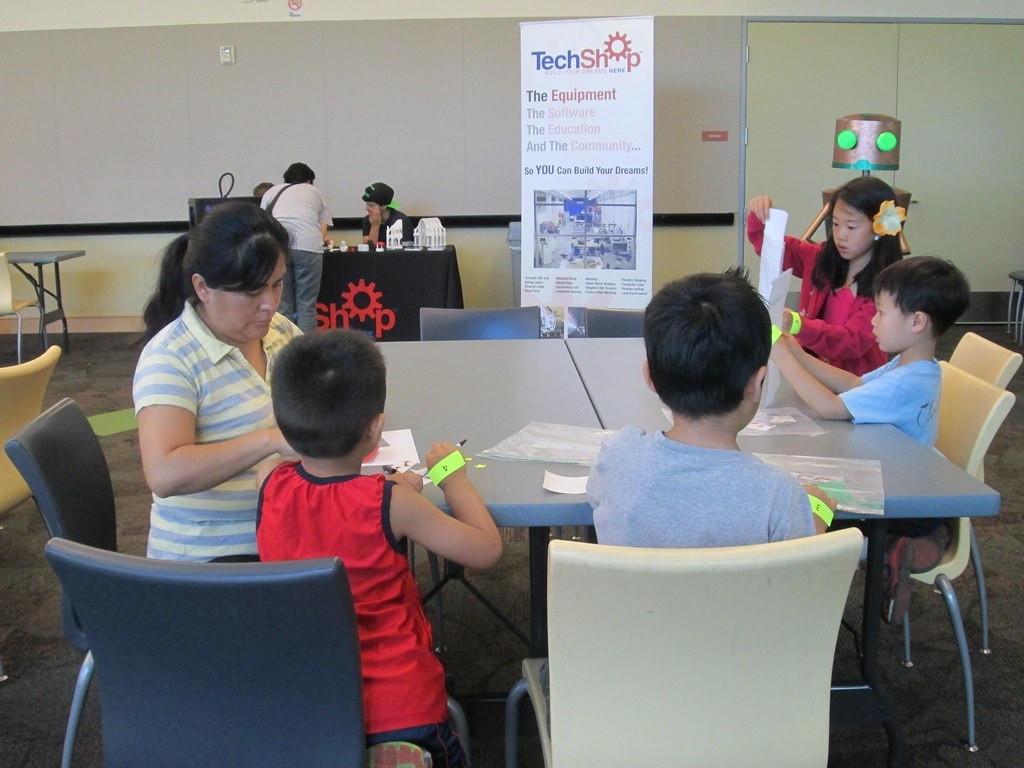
[86, 407, 138, 437]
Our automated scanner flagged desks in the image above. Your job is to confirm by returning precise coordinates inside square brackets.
[374, 340, 604, 741]
[293, 245, 464, 342]
[566, 337, 1001, 768]
[9, 250, 86, 354]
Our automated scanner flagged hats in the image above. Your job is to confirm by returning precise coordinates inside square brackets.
[362, 183, 394, 206]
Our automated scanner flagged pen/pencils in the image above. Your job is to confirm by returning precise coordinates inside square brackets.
[419, 437, 469, 485]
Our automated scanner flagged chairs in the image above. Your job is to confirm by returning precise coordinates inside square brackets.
[887, 331, 1024, 657]
[0, 251, 45, 365]
[420, 306, 542, 341]
[0, 346, 61, 539]
[1008, 270, 1024, 346]
[503, 527, 863, 768]
[908, 359, 1017, 751]
[4, 397, 119, 768]
[584, 309, 646, 338]
[44, 536, 470, 768]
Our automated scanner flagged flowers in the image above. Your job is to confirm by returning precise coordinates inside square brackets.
[872, 201, 908, 236]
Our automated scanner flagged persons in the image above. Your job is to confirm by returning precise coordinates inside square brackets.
[256, 327, 502, 768]
[260, 162, 334, 333]
[586, 273, 839, 548]
[253, 182, 274, 199]
[747, 176, 903, 376]
[771, 255, 970, 627]
[131, 200, 304, 563]
[361, 182, 414, 245]
